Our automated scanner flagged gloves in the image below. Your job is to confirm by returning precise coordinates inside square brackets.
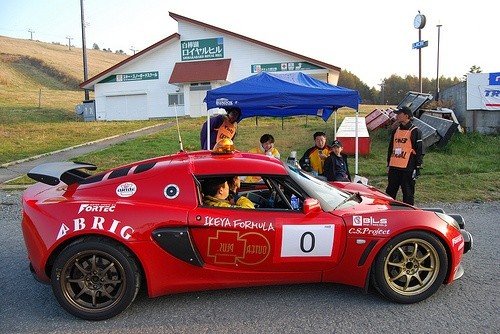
[412, 165, 423, 180]
[387, 167, 389, 173]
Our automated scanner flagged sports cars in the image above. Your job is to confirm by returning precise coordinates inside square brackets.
[16, 139, 474, 322]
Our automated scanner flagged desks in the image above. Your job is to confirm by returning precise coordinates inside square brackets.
[335, 116, 370, 155]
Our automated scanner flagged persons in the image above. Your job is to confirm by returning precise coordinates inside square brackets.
[199, 106, 241, 150]
[299, 131, 351, 182]
[386, 106, 425, 206]
[201, 176, 255, 209]
[239, 134, 280, 184]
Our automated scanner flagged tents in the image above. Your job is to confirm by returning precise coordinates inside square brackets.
[203, 71, 362, 175]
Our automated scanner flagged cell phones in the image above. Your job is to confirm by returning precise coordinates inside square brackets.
[319, 150, 322, 154]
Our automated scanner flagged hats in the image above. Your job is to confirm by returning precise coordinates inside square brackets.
[393, 106, 412, 116]
[331, 140, 342, 148]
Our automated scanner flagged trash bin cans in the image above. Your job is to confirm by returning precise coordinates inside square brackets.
[82, 99, 96, 122]
